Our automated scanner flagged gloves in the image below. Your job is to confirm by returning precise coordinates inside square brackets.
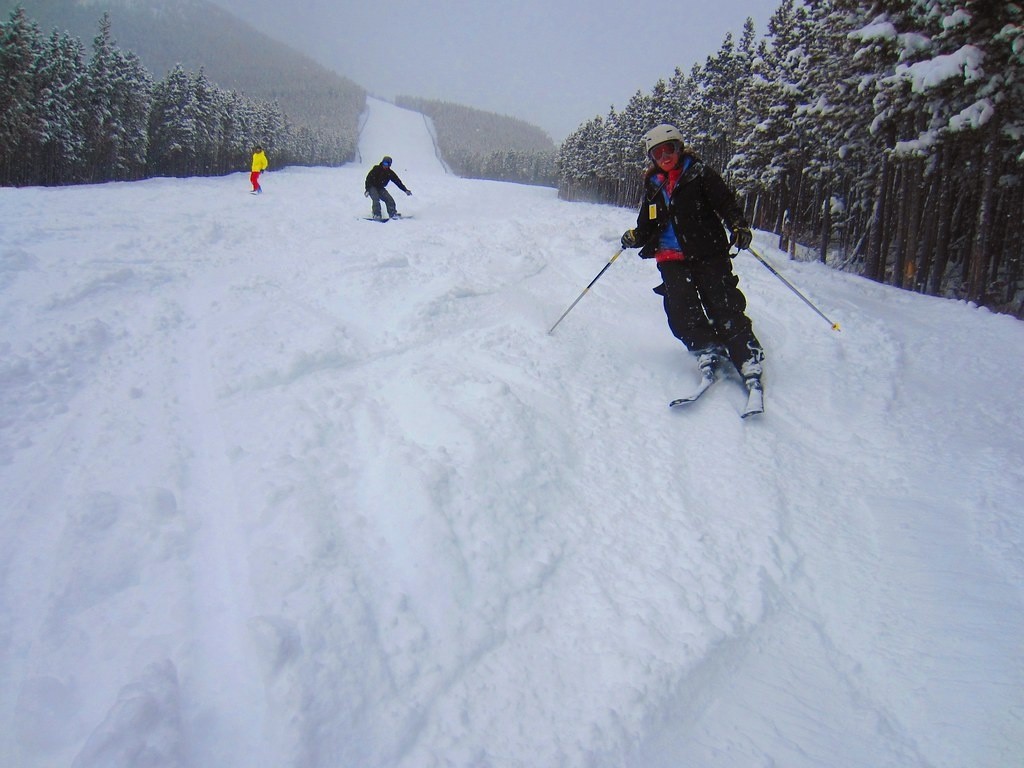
[405, 190, 413, 196]
[723, 208, 752, 250]
[620, 228, 640, 248]
[364, 191, 370, 197]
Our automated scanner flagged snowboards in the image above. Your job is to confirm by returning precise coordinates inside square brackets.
[249, 187, 261, 197]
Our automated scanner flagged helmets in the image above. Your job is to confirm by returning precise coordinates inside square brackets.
[645, 124, 684, 154]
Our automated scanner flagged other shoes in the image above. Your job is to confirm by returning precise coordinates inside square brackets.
[389, 213, 401, 219]
[739, 355, 763, 380]
[695, 352, 720, 373]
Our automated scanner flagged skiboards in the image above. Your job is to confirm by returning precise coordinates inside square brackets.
[664, 359, 767, 420]
[359, 215, 414, 223]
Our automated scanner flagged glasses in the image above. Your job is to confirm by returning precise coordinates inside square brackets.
[647, 141, 680, 162]
[383, 161, 392, 167]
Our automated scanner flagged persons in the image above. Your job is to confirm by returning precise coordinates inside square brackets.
[619, 123, 766, 379]
[248, 145, 268, 195]
[363, 155, 413, 221]
